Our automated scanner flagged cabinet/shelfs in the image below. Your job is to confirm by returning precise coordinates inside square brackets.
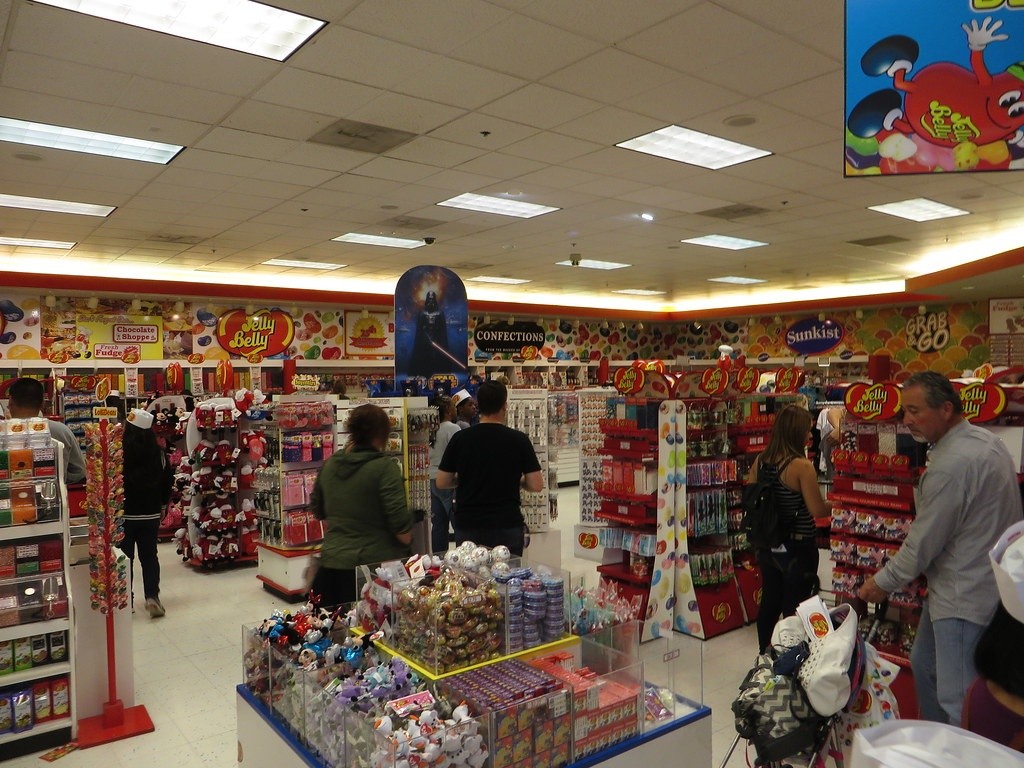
[596, 399, 675, 643]
[824, 491, 925, 667]
[0, 440, 78, 745]
[150, 395, 550, 604]
[674, 393, 819, 648]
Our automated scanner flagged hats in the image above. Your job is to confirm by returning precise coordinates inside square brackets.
[451, 388, 472, 408]
[125, 407, 154, 429]
[988, 520, 1024, 624]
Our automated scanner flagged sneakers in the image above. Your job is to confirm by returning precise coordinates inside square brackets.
[144, 594, 166, 618]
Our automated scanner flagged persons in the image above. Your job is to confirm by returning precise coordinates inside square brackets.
[860, 372, 1024, 729]
[309, 404, 414, 602]
[824, 408, 843, 444]
[749, 406, 833, 655]
[430, 381, 545, 555]
[8, 377, 84, 483]
[121, 410, 165, 615]
[962, 601, 1024, 755]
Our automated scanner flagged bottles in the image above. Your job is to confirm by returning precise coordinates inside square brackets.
[262, 437, 279, 460]
[253, 486, 281, 545]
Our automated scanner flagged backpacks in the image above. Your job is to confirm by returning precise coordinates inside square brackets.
[739, 456, 807, 553]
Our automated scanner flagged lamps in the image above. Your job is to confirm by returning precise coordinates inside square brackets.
[537, 317, 543, 326]
[694, 320, 701, 329]
[573, 320, 579, 327]
[749, 315, 755, 325]
[819, 311, 825, 320]
[856, 310, 863, 318]
[919, 305, 926, 314]
[637, 321, 643, 329]
[619, 321, 624, 329]
[484, 313, 491, 324]
[775, 314, 782, 323]
[554, 318, 560, 326]
[508, 315, 514, 325]
[603, 320, 608, 328]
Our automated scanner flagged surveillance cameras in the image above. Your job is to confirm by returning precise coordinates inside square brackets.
[425, 238, 435, 245]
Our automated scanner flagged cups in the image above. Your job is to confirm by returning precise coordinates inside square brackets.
[343, 408, 404, 478]
[252, 465, 280, 486]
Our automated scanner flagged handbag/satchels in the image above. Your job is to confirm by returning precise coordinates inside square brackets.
[770, 596, 859, 717]
[826, 643, 902, 768]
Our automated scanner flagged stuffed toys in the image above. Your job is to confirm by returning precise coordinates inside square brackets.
[156, 388, 269, 563]
